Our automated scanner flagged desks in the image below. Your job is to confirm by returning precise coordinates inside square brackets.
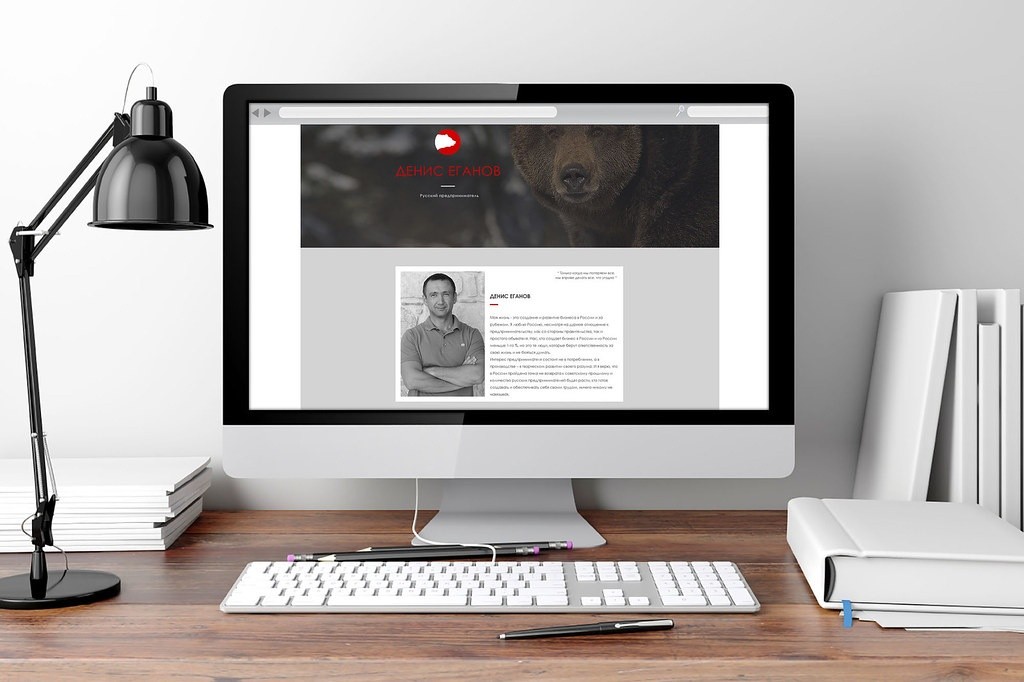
[0, 510, 1024, 682]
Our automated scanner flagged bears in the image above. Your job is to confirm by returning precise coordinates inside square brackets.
[511, 125, 719, 248]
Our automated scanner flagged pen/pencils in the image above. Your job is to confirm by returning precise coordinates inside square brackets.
[496, 617, 675, 639]
[287, 540, 574, 564]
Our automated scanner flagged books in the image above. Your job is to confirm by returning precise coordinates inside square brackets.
[0, 456, 213, 554]
[786, 288, 1024, 633]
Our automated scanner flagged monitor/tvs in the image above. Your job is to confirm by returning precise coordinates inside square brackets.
[218, 82, 797, 548]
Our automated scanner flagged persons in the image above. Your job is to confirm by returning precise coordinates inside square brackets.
[401, 273, 485, 395]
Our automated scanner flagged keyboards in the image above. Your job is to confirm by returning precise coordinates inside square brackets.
[220, 559, 762, 613]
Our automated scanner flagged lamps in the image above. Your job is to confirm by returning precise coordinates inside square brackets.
[0, 61, 216, 609]
